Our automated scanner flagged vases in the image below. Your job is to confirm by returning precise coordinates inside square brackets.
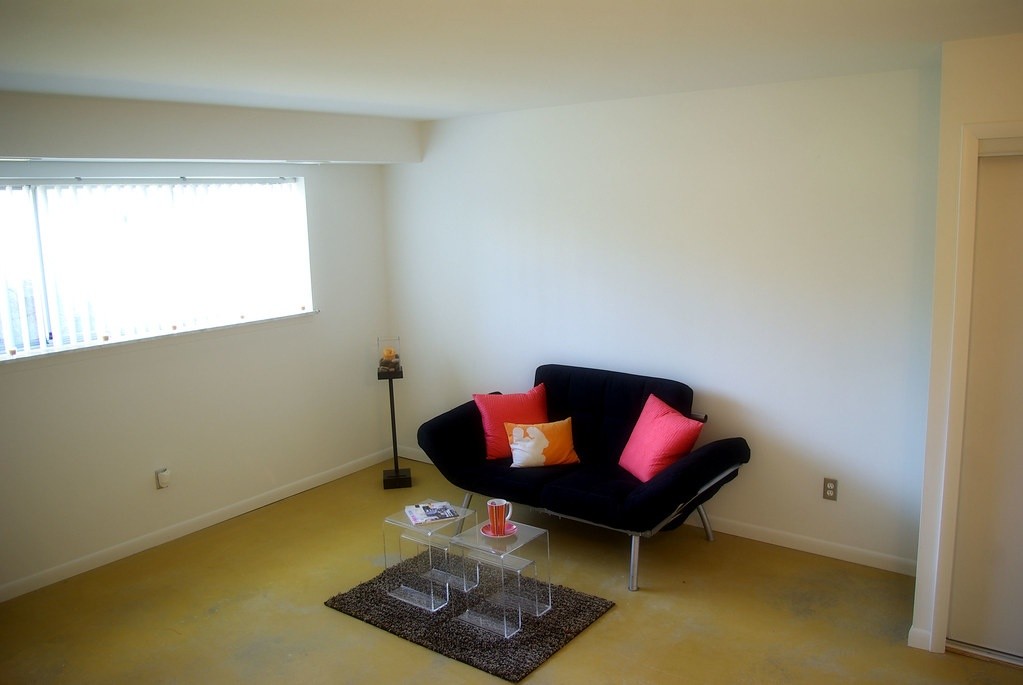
[376, 334, 401, 372]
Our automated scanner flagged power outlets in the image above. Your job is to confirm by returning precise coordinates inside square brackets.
[825, 476, 838, 502]
[154, 468, 170, 490]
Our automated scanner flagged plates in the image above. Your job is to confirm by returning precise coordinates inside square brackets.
[480, 522, 518, 538]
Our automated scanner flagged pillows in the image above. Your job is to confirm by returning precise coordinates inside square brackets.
[502, 415, 582, 470]
[619, 394, 705, 483]
[471, 379, 548, 463]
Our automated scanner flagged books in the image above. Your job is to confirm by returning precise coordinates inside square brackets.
[404, 501, 459, 526]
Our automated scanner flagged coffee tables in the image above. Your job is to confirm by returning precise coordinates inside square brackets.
[379, 496, 554, 640]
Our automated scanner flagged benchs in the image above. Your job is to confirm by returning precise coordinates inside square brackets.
[411, 362, 752, 593]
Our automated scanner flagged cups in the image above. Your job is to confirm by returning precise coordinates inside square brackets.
[487, 499, 513, 536]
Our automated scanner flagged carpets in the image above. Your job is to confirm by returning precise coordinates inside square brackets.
[324, 542, 617, 684]
